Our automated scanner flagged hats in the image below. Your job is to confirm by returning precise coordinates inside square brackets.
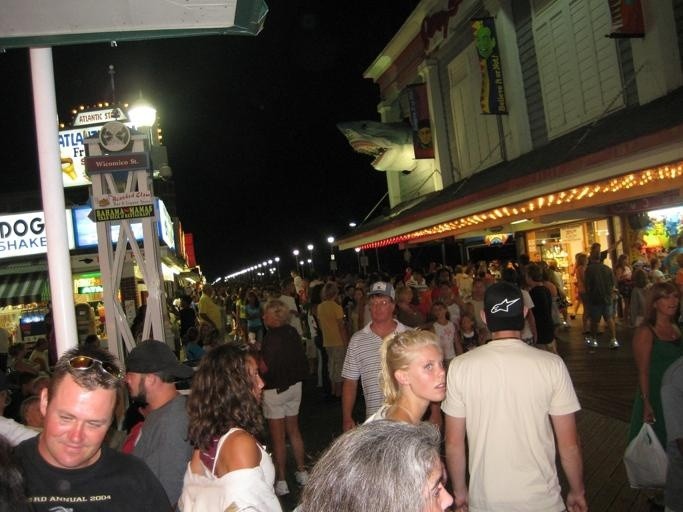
[367, 282, 396, 301]
[125, 340, 195, 379]
[483, 282, 525, 333]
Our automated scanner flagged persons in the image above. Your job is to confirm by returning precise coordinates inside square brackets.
[415, 119, 433, 149]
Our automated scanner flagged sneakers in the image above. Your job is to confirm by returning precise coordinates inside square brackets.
[275, 480, 290, 496]
[608, 339, 620, 349]
[293, 471, 309, 485]
[584, 335, 599, 348]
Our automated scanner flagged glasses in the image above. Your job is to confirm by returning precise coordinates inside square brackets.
[65, 356, 120, 381]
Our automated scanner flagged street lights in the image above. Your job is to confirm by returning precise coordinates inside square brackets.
[210, 221, 362, 286]
[125, 84, 158, 176]
